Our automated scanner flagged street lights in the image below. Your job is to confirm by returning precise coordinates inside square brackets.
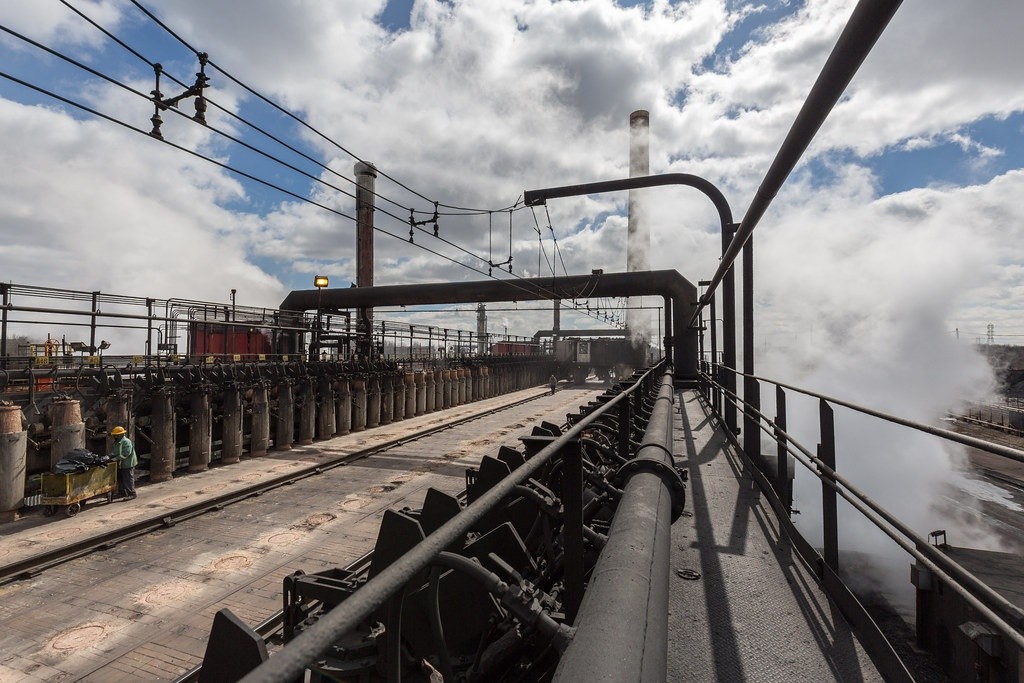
[70, 341, 111, 385]
[313, 274, 328, 360]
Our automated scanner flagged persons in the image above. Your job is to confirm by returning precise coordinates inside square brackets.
[548, 374, 557, 394]
[100, 425, 138, 501]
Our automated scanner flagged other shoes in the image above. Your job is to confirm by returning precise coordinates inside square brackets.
[123, 494, 137, 500]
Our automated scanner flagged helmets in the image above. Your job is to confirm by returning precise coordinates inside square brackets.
[111, 426, 127, 435]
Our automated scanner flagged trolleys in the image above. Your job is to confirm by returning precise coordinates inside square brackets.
[41, 459, 119, 516]
